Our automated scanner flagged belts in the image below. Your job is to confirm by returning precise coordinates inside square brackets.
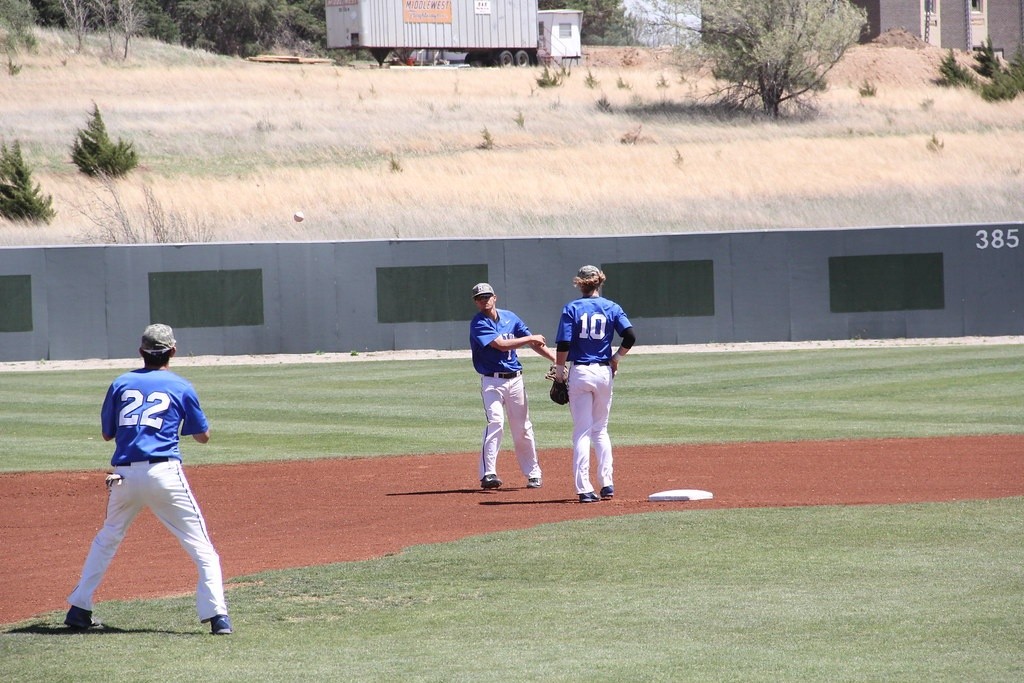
[574, 360, 610, 367]
[484, 369, 523, 379]
[117, 456, 168, 467]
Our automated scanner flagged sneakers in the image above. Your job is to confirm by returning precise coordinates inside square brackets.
[211, 615, 232, 634]
[579, 492, 601, 502]
[600, 486, 614, 500]
[527, 478, 542, 488]
[482, 474, 502, 489]
[65, 604, 104, 629]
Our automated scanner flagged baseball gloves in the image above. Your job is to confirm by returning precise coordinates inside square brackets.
[550, 381, 569, 405]
[545, 364, 569, 383]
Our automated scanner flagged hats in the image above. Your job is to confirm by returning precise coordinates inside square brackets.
[472, 283, 494, 298]
[141, 324, 177, 355]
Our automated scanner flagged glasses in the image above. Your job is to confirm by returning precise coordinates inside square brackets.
[475, 294, 493, 300]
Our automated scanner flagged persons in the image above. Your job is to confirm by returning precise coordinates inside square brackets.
[64, 322, 233, 635]
[546, 265, 635, 503]
[469, 282, 567, 489]
